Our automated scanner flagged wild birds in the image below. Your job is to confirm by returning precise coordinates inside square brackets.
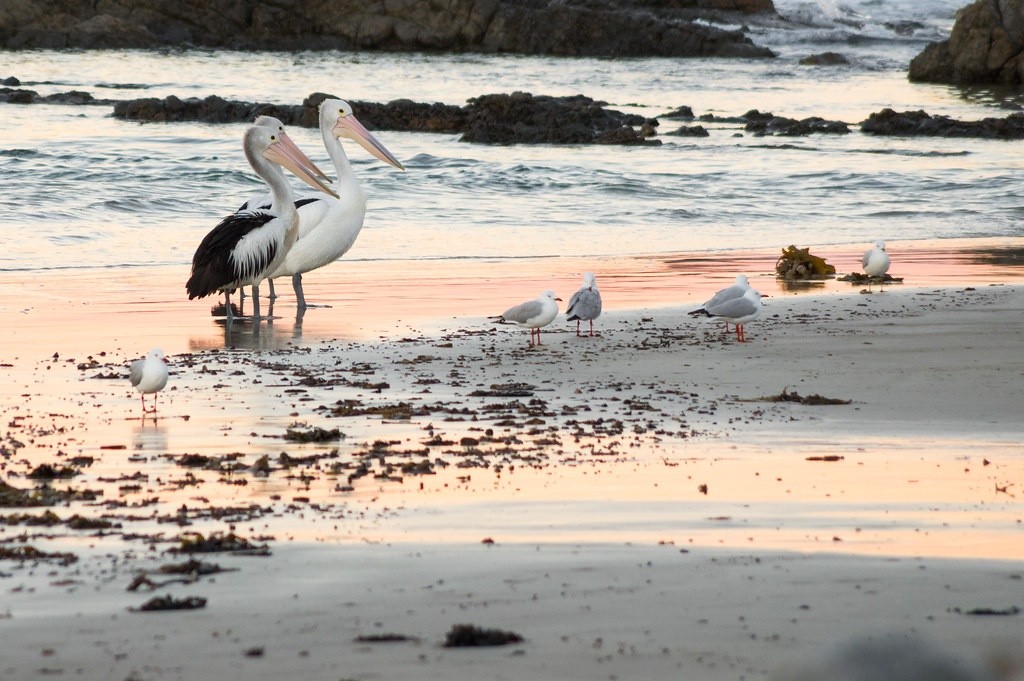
[486, 290, 563, 347]
[128, 347, 171, 414]
[687, 274, 770, 343]
[184, 97, 406, 335]
[564, 270, 602, 337]
[862, 241, 891, 293]
